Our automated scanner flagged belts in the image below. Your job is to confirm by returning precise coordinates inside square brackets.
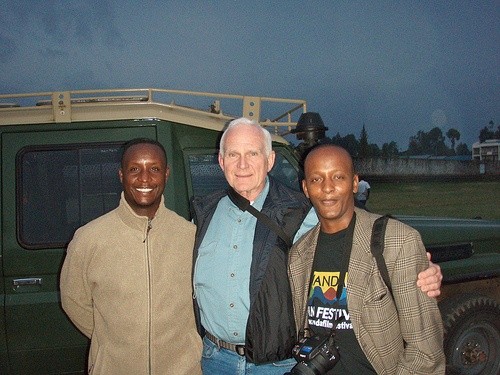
[205, 330, 247, 357]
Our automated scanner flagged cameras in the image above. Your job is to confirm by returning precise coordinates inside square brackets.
[283, 334, 340, 375]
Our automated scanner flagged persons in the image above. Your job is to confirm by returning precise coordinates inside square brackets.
[59, 138, 204, 375]
[286, 143, 446, 375]
[355, 176, 371, 206]
[190, 118, 443, 375]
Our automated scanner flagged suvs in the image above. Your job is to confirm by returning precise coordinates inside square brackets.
[0, 87, 500, 374]
